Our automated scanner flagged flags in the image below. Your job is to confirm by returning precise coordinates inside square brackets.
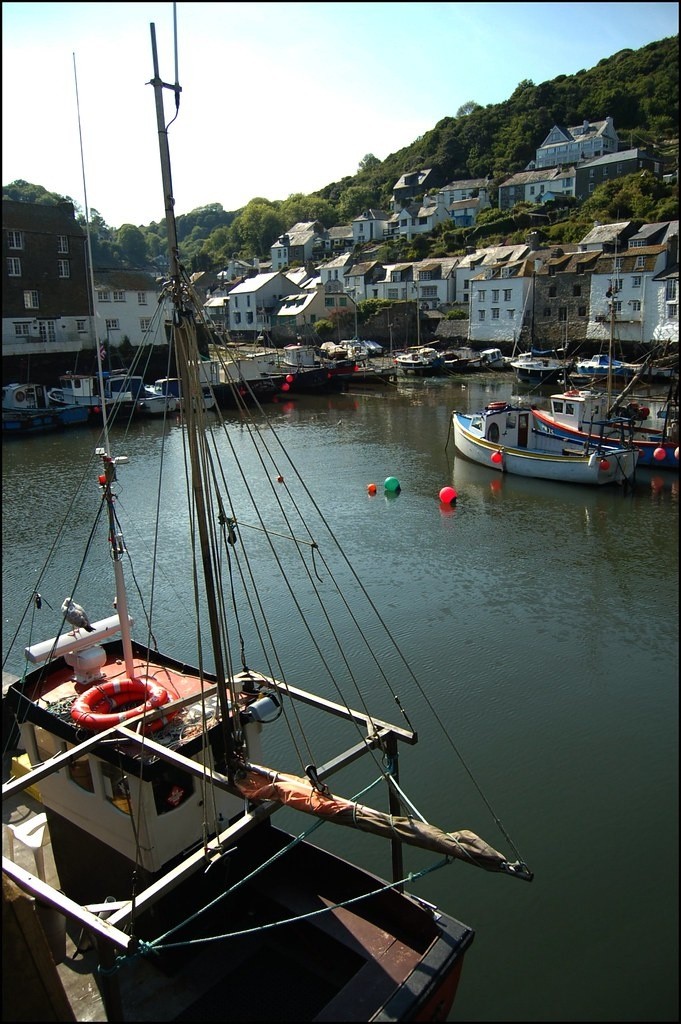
[99, 338, 106, 362]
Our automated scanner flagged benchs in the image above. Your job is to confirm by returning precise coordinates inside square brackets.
[563, 448, 584, 456]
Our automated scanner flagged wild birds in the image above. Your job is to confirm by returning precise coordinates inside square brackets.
[60, 597, 96, 636]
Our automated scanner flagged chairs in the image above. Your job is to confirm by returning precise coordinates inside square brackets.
[8, 813, 52, 883]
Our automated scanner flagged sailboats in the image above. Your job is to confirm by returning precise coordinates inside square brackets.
[1, 0, 681, 1024]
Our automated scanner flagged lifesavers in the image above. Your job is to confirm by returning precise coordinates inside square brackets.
[564, 390, 579, 397]
[69, 678, 180, 736]
[488, 401, 507, 409]
